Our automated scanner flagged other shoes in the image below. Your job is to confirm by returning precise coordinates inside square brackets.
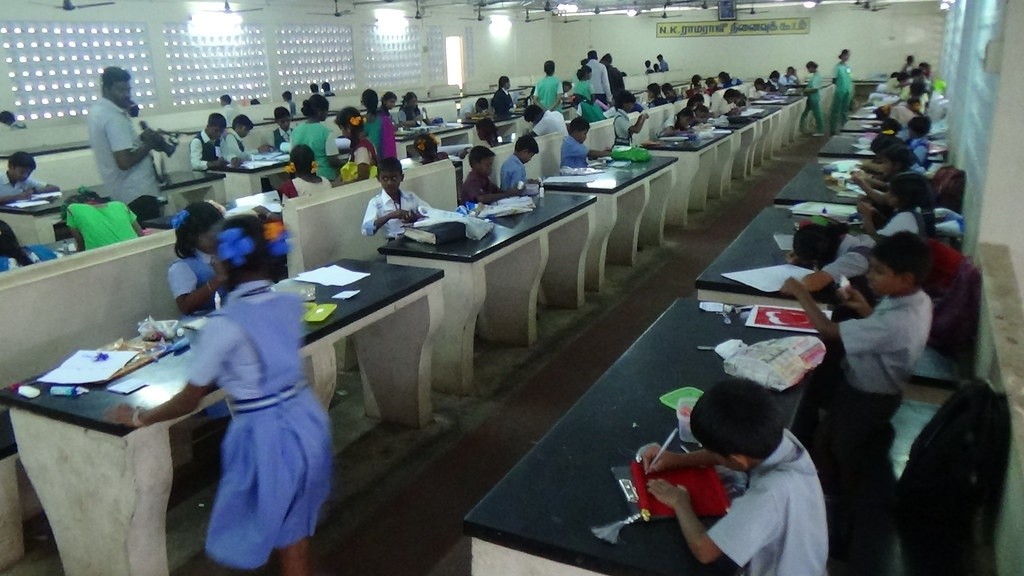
[812, 132, 824, 136]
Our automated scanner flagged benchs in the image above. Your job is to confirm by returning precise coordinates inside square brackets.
[845, 321, 962, 576]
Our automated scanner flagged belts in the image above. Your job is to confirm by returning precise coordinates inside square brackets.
[616, 138, 629, 143]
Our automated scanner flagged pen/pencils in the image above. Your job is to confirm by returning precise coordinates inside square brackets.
[20, 187, 25, 191]
[643, 429, 678, 479]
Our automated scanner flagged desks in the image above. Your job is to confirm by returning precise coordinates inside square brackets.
[1, 74, 903, 576]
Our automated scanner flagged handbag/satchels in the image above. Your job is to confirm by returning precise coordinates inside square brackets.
[340, 159, 377, 180]
[471, 195, 535, 217]
[611, 144, 650, 162]
[400, 221, 465, 246]
[417, 205, 493, 240]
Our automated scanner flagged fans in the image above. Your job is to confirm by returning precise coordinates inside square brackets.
[845, 0, 892, 12]
[30, 0, 769, 22]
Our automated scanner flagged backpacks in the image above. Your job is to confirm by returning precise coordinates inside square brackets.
[886, 379, 1007, 526]
[927, 254, 979, 356]
[930, 166, 964, 209]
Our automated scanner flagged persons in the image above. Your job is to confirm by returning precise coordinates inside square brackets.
[220, 94, 234, 112]
[86, 66, 163, 226]
[0, 110, 28, 130]
[282, 91, 296, 115]
[188, 112, 245, 171]
[322, 82, 335, 97]
[272, 106, 294, 152]
[59, 189, 144, 251]
[461, 146, 527, 206]
[269, 87, 431, 199]
[0, 151, 60, 206]
[310, 84, 319, 93]
[107, 203, 335, 575]
[412, 134, 448, 165]
[357, 157, 432, 241]
[642, 55, 936, 576]
[0, 220, 59, 271]
[459, 96, 490, 120]
[493, 49, 855, 192]
[226, 115, 272, 159]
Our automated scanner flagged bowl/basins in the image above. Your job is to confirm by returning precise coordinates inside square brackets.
[677, 398, 697, 441]
[137, 320, 178, 340]
